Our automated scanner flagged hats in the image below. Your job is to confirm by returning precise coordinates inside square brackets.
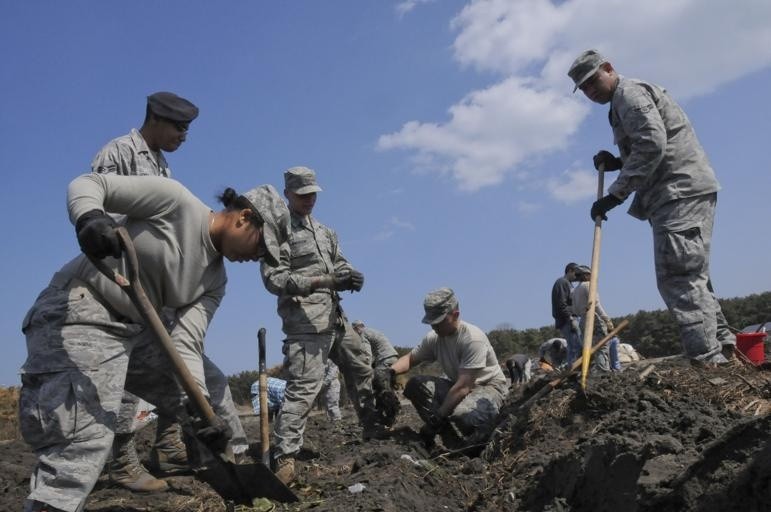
[145, 91, 200, 123]
[421, 287, 459, 325]
[351, 320, 365, 328]
[241, 183, 293, 268]
[574, 265, 594, 276]
[568, 48, 608, 95]
[284, 166, 322, 196]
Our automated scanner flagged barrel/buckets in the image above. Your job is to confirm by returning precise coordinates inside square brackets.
[735, 332, 767, 364]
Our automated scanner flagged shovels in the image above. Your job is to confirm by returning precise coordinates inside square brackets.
[487, 319, 630, 442]
[92, 227, 300, 508]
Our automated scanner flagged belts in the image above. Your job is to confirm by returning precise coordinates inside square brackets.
[49, 271, 132, 326]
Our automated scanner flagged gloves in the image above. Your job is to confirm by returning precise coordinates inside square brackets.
[75, 209, 126, 262]
[593, 150, 622, 171]
[349, 268, 364, 292]
[317, 270, 354, 291]
[372, 368, 393, 390]
[200, 413, 233, 446]
[591, 194, 621, 222]
[418, 414, 443, 447]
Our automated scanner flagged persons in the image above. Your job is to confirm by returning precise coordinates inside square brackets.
[567, 50, 736, 365]
[258, 167, 399, 481]
[373, 286, 510, 452]
[16, 91, 291, 512]
[505, 263, 622, 392]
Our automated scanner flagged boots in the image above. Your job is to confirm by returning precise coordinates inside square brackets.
[108, 432, 169, 494]
[149, 417, 199, 475]
[362, 423, 390, 440]
[273, 453, 298, 489]
